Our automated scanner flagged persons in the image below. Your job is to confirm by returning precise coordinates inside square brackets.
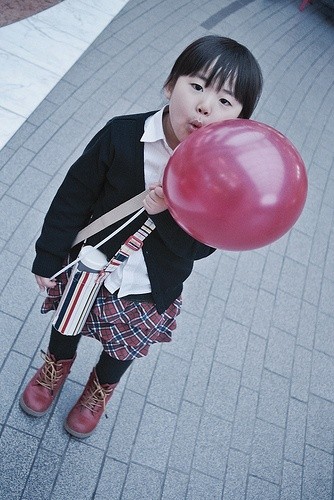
[20, 29, 265, 441]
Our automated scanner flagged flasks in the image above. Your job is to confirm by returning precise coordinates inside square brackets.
[52, 244, 109, 337]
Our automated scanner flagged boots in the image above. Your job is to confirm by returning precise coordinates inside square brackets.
[19, 348, 78, 417]
[64, 367, 120, 437]
